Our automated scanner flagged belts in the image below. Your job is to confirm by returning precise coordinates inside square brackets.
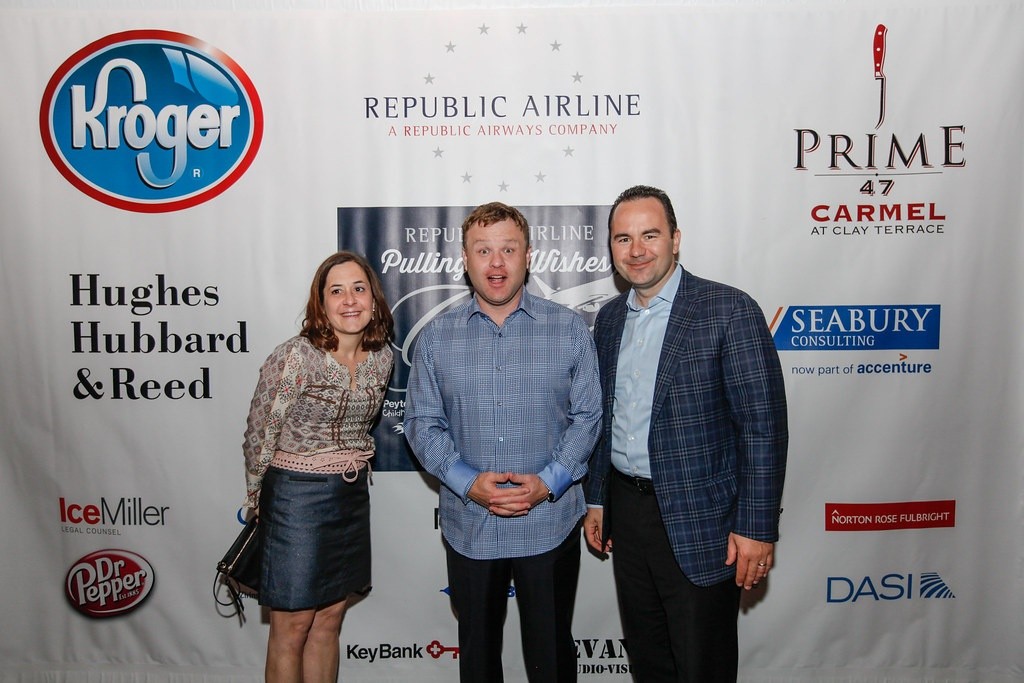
[615, 472, 653, 492]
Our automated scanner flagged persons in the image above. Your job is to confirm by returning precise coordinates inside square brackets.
[583, 186, 789, 683]
[404, 202, 604, 683]
[243, 250, 395, 683]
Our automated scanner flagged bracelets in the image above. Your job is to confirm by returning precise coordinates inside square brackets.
[538, 478, 554, 502]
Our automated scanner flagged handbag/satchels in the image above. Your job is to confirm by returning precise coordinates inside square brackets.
[215, 516, 262, 618]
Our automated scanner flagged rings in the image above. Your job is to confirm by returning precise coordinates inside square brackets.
[758, 563, 767, 566]
